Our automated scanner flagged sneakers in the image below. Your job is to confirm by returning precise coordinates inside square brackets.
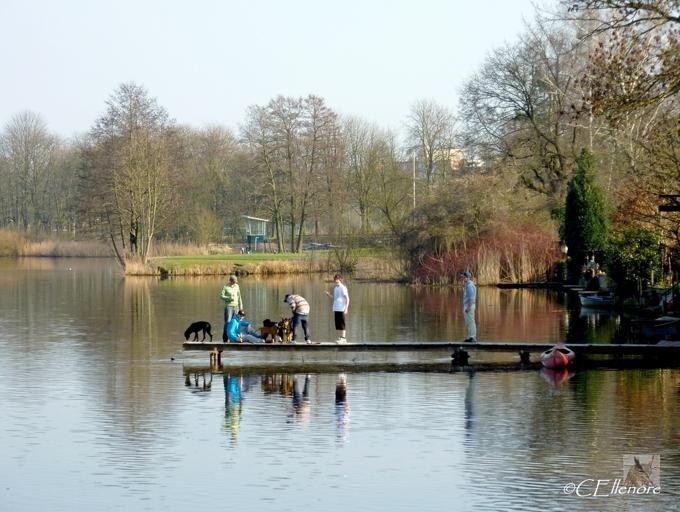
[336, 337, 346, 343]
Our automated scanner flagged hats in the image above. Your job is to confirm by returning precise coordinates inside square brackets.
[239, 310, 245, 316]
[464, 271, 471, 277]
[283, 295, 289, 302]
[229, 275, 238, 282]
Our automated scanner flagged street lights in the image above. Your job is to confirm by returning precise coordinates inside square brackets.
[560, 240, 569, 283]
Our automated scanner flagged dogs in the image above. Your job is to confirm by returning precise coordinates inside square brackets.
[261, 317, 294, 343]
[184, 321, 213, 342]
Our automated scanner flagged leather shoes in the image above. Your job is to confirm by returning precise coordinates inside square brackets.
[465, 338, 476, 343]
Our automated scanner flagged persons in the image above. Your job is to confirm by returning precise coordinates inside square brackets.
[461, 271, 478, 343]
[219, 372, 351, 453]
[220, 275, 244, 342]
[324, 273, 350, 343]
[462, 370, 478, 438]
[284, 294, 311, 343]
[227, 309, 266, 343]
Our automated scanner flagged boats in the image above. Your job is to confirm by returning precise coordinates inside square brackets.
[578, 294, 615, 306]
[630, 315, 680, 329]
[578, 307, 611, 319]
[539, 340, 577, 370]
[539, 365, 577, 389]
